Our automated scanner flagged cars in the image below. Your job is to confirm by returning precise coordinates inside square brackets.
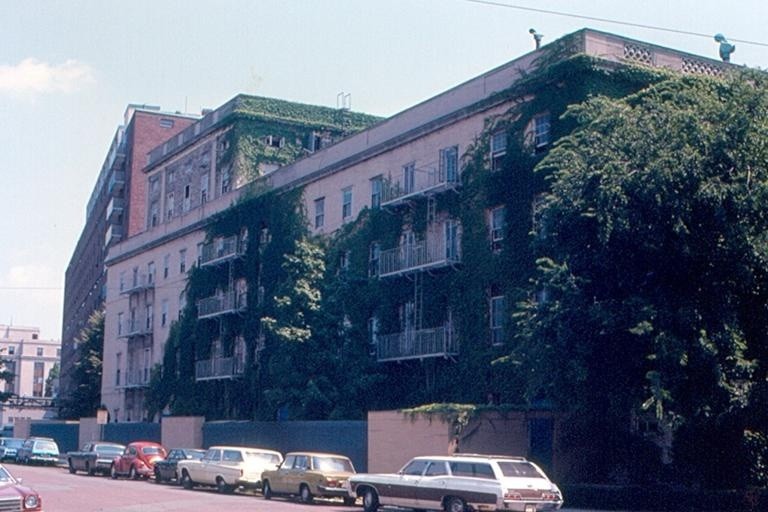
[178, 445, 284, 494]
[110, 441, 169, 479]
[66, 441, 127, 476]
[262, 451, 357, 504]
[0, 436, 60, 465]
[349, 454, 564, 512]
[0, 465, 41, 512]
[155, 448, 228, 489]
